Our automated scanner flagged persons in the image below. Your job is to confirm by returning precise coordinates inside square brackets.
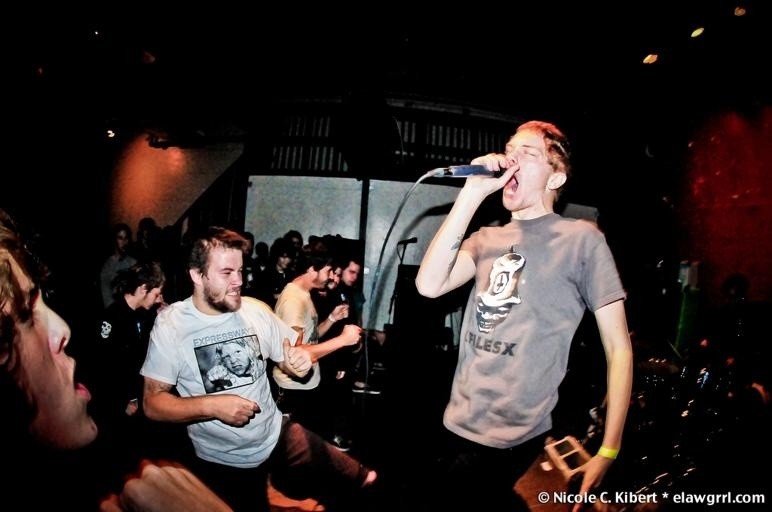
[414, 120, 634, 511]
[2, 211, 379, 512]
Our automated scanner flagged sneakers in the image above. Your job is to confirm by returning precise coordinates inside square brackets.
[333, 436, 352, 452]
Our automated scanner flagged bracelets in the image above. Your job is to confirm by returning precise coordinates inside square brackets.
[596, 446, 620, 460]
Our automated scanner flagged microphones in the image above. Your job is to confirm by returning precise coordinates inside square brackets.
[397, 237, 418, 245]
[444, 164, 507, 179]
